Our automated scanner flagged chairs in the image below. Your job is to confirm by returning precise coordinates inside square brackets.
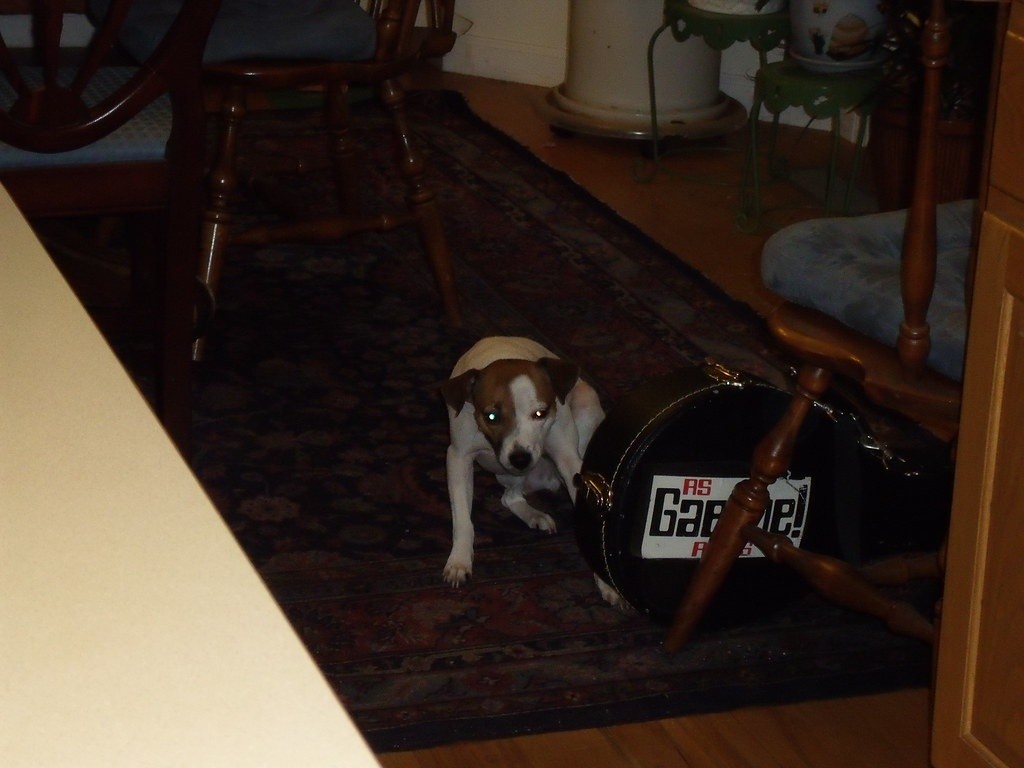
[666, 0, 1024, 672]
[0, 0, 472, 354]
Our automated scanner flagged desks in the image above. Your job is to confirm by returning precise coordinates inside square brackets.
[0, 182, 383, 768]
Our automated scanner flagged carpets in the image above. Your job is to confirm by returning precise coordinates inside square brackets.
[32, 96, 956, 759]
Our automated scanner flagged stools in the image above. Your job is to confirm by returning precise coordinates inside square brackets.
[737, 60, 883, 240]
[629, 0, 789, 197]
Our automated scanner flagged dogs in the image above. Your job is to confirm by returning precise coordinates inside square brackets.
[441, 335, 606, 590]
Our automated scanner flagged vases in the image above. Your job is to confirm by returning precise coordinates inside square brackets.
[554, 0, 727, 127]
[786, 0, 890, 75]
